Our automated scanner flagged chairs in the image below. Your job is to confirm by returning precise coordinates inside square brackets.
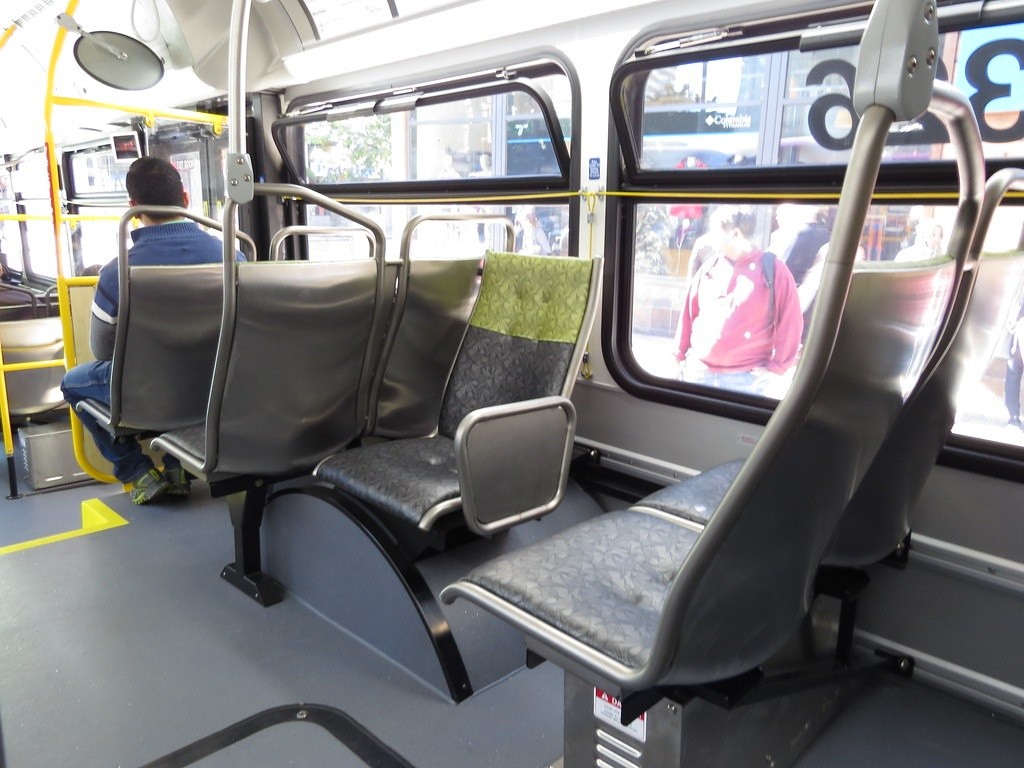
[148, 182, 385, 605]
[313, 249, 607, 535]
[636, 165, 1024, 677]
[364, 214, 519, 430]
[75, 204, 258, 479]
[437, 79, 987, 744]
[0, 284, 72, 435]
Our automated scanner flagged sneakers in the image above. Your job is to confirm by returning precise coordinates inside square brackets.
[127, 471, 167, 506]
[159, 464, 191, 496]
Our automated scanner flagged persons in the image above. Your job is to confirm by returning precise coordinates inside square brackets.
[668, 205, 804, 399]
[765, 201, 950, 348]
[435, 155, 459, 177]
[687, 234, 710, 277]
[1005, 303, 1024, 434]
[477, 155, 492, 176]
[513, 212, 549, 255]
[58, 156, 247, 504]
